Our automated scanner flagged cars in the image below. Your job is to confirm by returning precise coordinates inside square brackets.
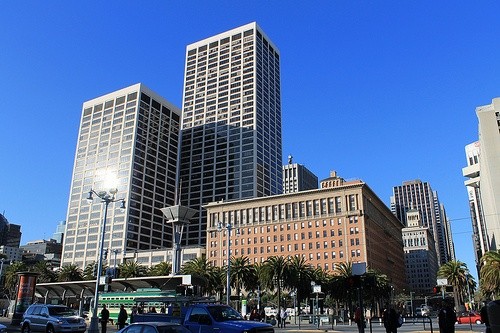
[19, 303, 87, 333]
[455, 310, 483, 324]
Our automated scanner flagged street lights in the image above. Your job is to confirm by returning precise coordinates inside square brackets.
[87, 188, 126, 333]
[217, 220, 240, 305]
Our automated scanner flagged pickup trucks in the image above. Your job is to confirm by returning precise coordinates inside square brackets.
[130, 302, 275, 333]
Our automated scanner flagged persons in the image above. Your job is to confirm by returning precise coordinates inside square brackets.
[2, 307, 6, 317]
[100, 303, 109, 333]
[445, 306, 455, 333]
[354, 307, 362, 331]
[243, 307, 288, 329]
[74, 303, 79, 315]
[214, 305, 234, 321]
[472, 303, 477, 311]
[362, 311, 366, 328]
[129, 305, 165, 323]
[486, 298, 500, 333]
[118, 305, 128, 331]
[438, 308, 447, 333]
[383, 302, 397, 333]
[480, 301, 492, 333]
